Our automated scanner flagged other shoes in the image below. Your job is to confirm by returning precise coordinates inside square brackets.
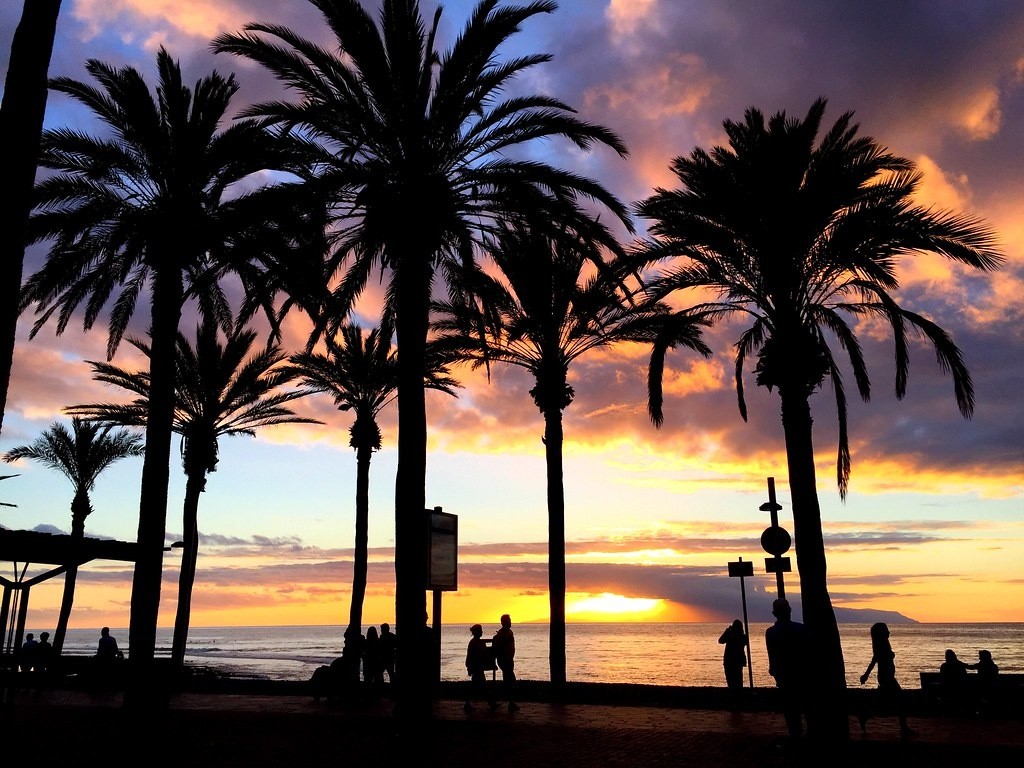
[858, 713, 868, 730]
[509, 702, 520, 711]
[491, 701, 503, 710]
[900, 726, 919, 738]
[463, 703, 476, 714]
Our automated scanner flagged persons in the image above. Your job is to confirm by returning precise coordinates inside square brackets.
[20, 632, 52, 672]
[492, 615, 520, 710]
[767, 599, 820, 738]
[719, 620, 748, 711]
[856, 623, 919, 737]
[464, 624, 504, 713]
[96, 628, 118, 658]
[940, 650, 1000, 727]
[344, 624, 396, 687]
